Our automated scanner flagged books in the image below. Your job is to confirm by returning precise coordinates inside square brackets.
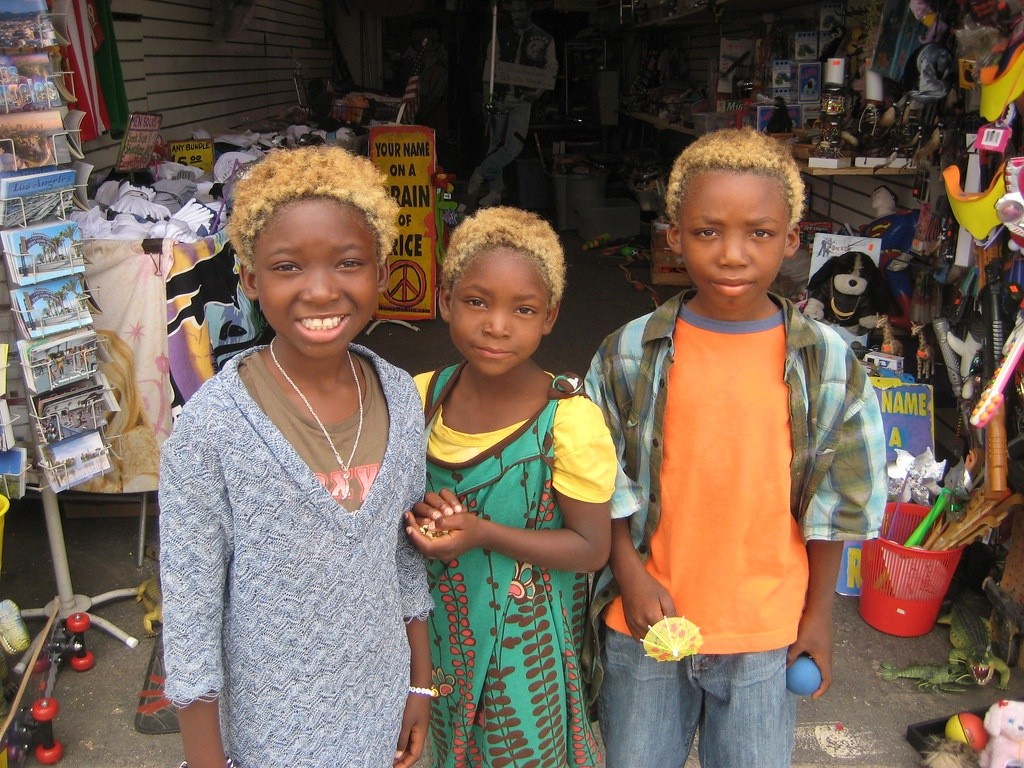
[0, 0, 121, 499]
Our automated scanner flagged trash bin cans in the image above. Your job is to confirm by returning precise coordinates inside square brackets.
[859, 501, 967, 639]
[550, 166, 608, 231]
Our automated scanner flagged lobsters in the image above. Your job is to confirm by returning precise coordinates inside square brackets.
[878, 661, 976, 697]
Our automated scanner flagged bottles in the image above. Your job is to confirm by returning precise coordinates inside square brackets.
[581, 233, 612, 250]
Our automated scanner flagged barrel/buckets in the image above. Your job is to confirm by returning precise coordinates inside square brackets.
[540, 168, 608, 231]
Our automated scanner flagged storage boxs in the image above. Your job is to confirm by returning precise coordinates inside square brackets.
[575, 197, 642, 243]
[690, 110, 737, 134]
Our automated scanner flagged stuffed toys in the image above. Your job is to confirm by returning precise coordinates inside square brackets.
[803, 252, 904, 327]
[922, 698, 1024, 768]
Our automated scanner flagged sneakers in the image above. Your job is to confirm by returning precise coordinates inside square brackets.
[468, 167, 503, 205]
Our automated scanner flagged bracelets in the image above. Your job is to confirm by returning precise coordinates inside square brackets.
[180, 756, 234, 768]
[409, 686, 441, 698]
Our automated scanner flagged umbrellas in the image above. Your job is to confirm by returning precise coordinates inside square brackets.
[640, 615, 704, 664]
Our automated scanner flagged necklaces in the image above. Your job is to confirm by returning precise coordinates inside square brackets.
[269, 336, 364, 478]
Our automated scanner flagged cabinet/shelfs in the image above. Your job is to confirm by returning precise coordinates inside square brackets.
[615, 0, 932, 233]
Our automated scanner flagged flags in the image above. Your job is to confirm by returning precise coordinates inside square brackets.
[401, 47, 424, 123]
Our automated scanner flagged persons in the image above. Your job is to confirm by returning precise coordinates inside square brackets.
[403, 206, 620, 767]
[860, 184, 919, 329]
[583, 125, 888, 768]
[159, 143, 442, 768]
[468, 0, 558, 205]
[932, 48, 956, 87]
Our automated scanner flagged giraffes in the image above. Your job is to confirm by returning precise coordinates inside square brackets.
[910, 321, 937, 384]
[876, 312, 902, 357]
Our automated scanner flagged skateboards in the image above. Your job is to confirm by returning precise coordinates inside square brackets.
[0, 597, 96, 768]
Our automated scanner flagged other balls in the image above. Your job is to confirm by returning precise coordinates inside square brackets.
[786, 656, 822, 695]
